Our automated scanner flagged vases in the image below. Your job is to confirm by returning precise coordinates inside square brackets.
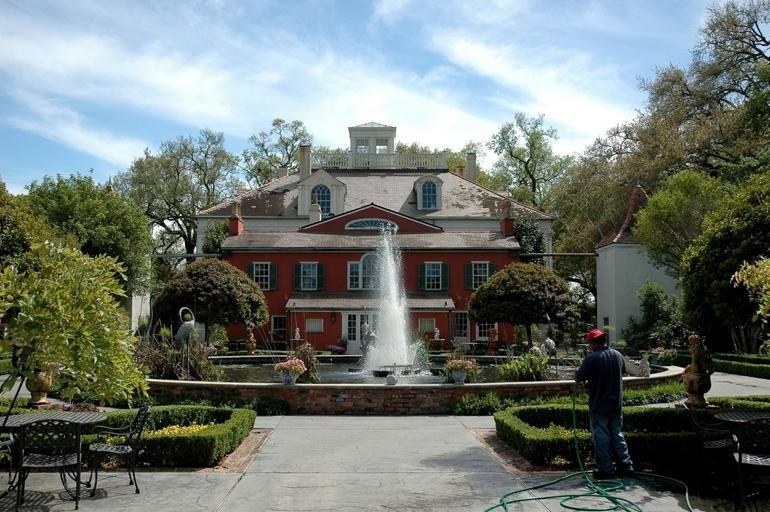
[451, 368, 467, 385]
[280, 370, 300, 385]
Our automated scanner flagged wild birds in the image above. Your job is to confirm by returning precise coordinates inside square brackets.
[174, 306, 197, 382]
[542, 311, 560, 381]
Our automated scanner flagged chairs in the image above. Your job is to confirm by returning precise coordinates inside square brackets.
[676, 399, 770, 512]
[1, 398, 149, 510]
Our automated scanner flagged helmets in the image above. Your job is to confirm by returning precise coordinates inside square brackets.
[585, 329, 609, 344]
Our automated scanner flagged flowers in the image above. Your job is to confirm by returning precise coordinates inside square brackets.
[440, 356, 482, 374]
[272, 358, 306, 378]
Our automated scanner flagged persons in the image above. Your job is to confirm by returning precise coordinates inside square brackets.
[574, 328, 635, 481]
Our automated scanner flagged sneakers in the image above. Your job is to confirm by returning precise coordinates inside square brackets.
[591, 464, 636, 480]
